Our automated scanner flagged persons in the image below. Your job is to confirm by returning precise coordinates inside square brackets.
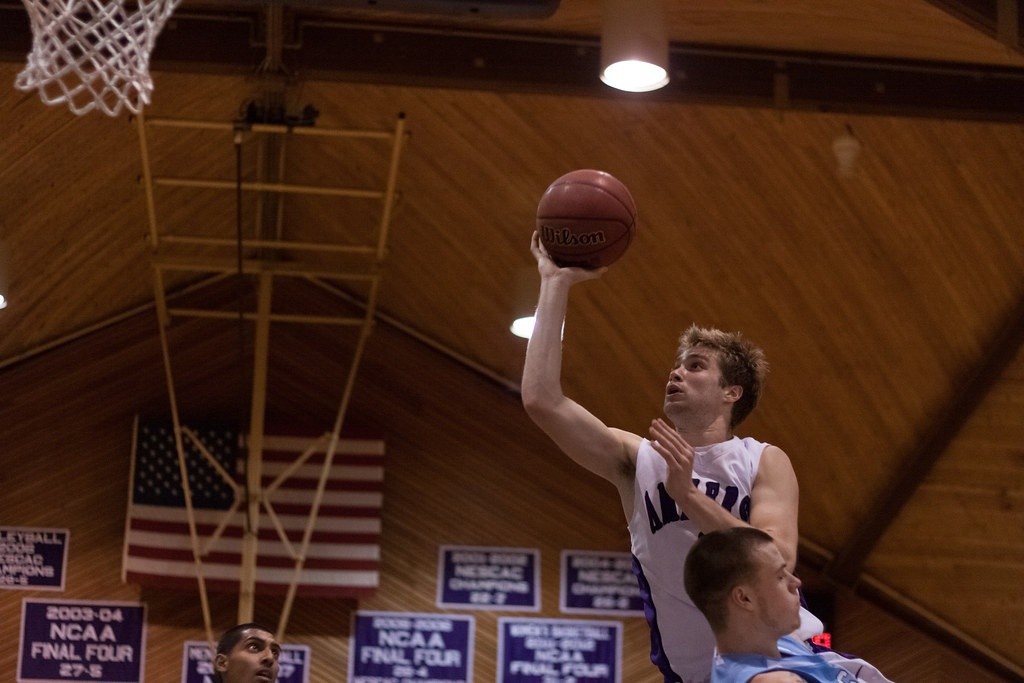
[214, 622, 283, 683]
[683, 526, 900, 683]
[519, 227, 829, 683]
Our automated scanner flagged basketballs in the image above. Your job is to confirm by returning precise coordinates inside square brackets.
[534, 167, 640, 271]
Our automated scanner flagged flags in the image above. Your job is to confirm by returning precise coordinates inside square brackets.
[119, 399, 387, 600]
